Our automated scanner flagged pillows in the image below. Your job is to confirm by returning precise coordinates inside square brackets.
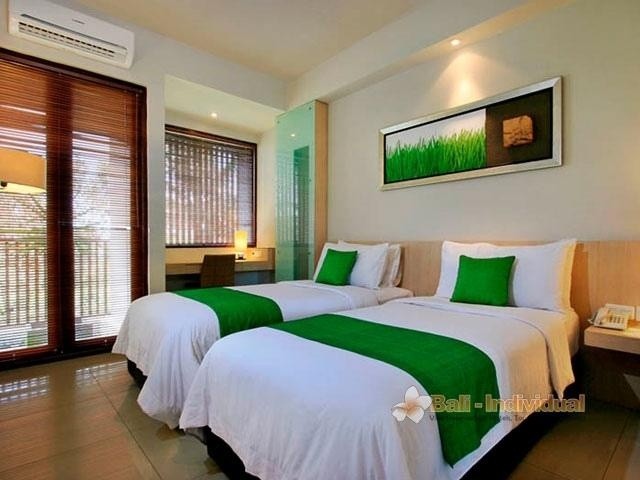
[313, 241, 386, 287]
[337, 238, 407, 288]
[316, 247, 356, 285]
[434, 238, 579, 314]
[449, 255, 516, 305]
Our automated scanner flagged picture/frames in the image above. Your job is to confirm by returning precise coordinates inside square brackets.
[376, 75, 562, 192]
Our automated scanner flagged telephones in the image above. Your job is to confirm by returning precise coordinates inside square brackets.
[592, 303, 634, 330]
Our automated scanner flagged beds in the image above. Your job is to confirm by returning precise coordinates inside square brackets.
[180, 223, 640, 480]
[112, 237, 440, 434]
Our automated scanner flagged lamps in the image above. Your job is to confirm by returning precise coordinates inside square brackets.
[0, 146, 46, 202]
[233, 230, 249, 262]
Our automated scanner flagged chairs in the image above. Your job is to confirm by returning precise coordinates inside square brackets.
[198, 253, 236, 286]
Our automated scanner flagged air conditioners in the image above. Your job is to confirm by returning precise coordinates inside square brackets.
[6, 1, 135, 68]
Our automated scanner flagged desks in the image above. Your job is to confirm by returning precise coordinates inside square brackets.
[165, 247, 277, 289]
[582, 319, 640, 355]
